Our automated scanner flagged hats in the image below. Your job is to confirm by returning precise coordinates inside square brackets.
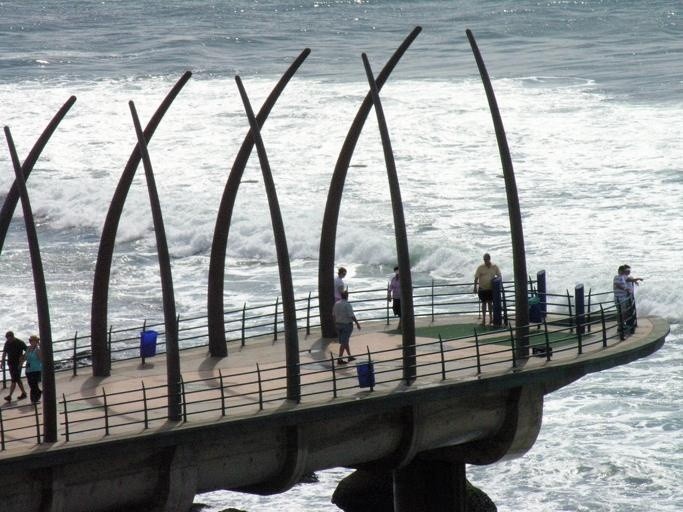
[618, 265, 631, 273]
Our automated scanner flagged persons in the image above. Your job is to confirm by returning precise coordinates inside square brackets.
[623, 263, 644, 294]
[386, 266, 402, 319]
[472, 253, 502, 327]
[612, 265, 630, 332]
[334, 266, 349, 301]
[17, 335, 42, 403]
[330, 290, 361, 365]
[0, 329, 28, 403]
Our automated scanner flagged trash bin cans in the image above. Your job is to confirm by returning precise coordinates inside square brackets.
[527, 297, 542, 324]
[357, 362, 376, 388]
[140, 331, 157, 357]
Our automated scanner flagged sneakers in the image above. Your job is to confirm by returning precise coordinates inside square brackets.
[17, 393, 27, 400]
[3, 395, 12, 402]
[337, 358, 348, 366]
[348, 357, 356, 362]
